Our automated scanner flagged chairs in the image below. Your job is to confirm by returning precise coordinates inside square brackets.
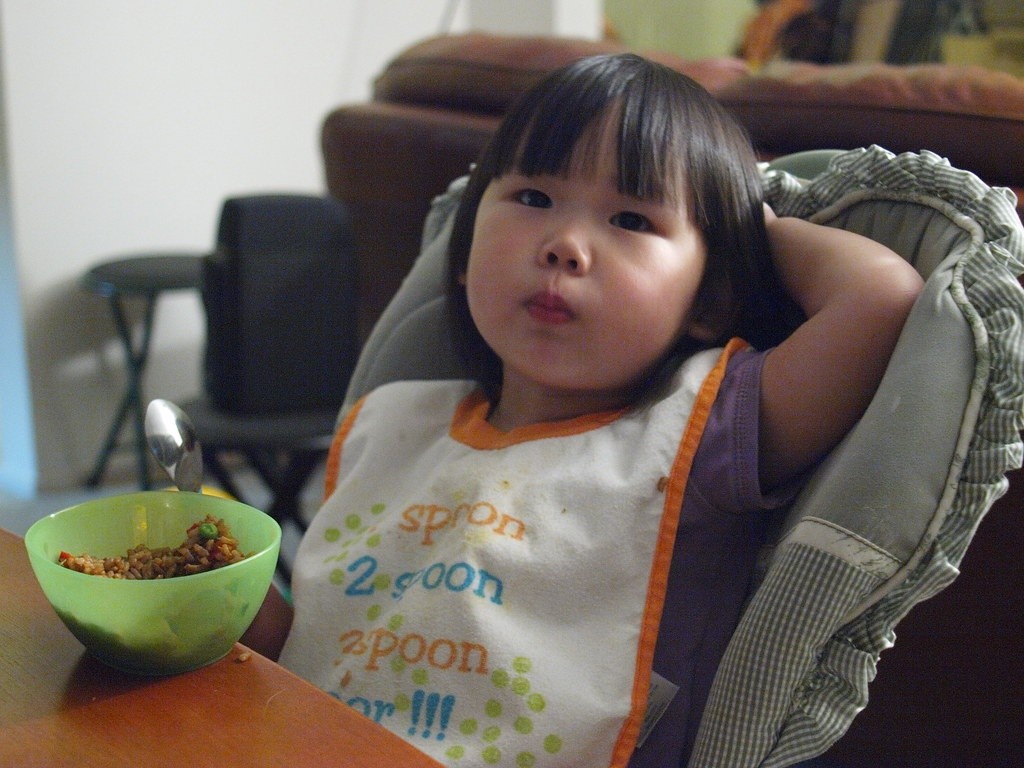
[0, 145, 1024, 768]
[168, 190, 358, 591]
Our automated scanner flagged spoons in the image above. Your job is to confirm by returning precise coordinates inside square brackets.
[144, 397, 203, 494]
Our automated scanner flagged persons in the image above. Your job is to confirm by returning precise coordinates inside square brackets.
[237, 52, 925, 768]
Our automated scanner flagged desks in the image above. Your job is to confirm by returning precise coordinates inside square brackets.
[80, 253, 213, 493]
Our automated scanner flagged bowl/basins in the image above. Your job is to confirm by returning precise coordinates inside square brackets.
[25, 492, 281, 676]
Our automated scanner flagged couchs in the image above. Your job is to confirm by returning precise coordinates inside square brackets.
[320, 32, 1024, 768]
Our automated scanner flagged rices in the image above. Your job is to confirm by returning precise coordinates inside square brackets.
[55, 513, 255, 579]
